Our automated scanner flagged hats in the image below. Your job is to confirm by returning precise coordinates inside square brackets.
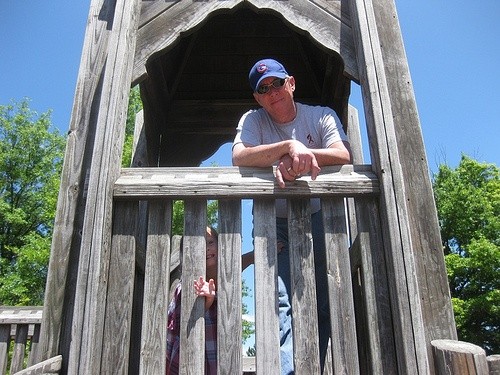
[248, 58, 290, 90]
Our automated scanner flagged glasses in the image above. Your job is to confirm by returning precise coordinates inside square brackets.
[257, 78, 286, 94]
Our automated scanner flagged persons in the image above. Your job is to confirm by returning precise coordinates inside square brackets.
[232, 58, 354, 375]
[166, 225, 285, 375]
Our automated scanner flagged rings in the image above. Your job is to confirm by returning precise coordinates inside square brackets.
[288, 168, 291, 171]
